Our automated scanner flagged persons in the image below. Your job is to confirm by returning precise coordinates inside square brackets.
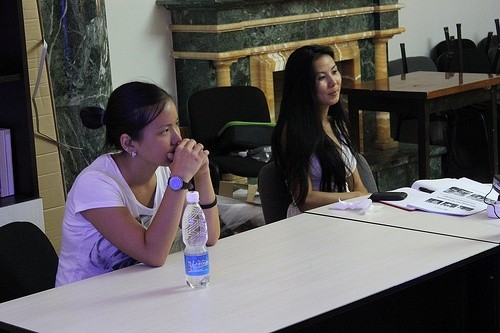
[54, 82, 220, 288]
[272, 44, 370, 218]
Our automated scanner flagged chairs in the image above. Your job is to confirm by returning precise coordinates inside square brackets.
[257, 150, 378, 223]
[188, 86, 270, 183]
[387, 17, 500, 178]
[0, 220, 58, 302]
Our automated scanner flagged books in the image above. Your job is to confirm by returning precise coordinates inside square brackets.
[378, 178, 459, 211]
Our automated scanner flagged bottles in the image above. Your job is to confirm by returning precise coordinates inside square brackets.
[181, 192, 209, 289]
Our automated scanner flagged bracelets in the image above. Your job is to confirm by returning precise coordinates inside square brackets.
[201, 196, 218, 209]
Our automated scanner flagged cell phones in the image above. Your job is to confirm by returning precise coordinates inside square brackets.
[369, 192, 408, 202]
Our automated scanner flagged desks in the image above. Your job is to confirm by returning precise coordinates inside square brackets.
[339, 71, 500, 182]
[0, 186, 500, 333]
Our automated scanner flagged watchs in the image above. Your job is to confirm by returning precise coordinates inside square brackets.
[167, 176, 193, 191]
